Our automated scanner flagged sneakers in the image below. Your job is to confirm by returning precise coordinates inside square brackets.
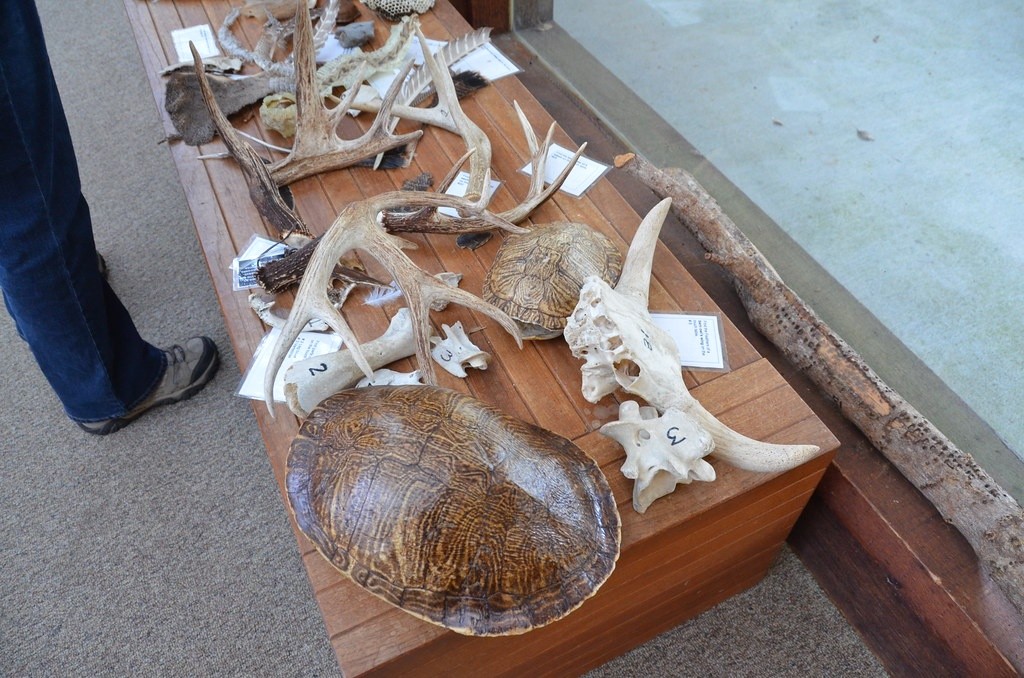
[74, 335, 219, 436]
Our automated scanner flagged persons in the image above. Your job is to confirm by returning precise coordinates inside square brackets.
[0, 0, 219, 436]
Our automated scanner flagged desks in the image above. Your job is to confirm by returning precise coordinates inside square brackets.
[124, 0, 841, 678]
